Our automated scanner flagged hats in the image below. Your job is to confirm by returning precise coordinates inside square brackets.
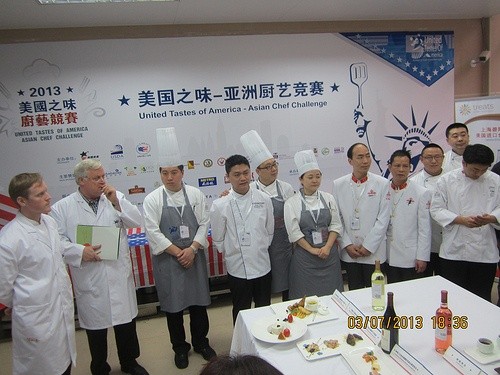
[240, 130, 274, 171]
[294, 149, 320, 178]
[156, 126, 184, 169]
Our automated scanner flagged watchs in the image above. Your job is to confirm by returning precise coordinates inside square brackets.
[190, 244, 199, 256]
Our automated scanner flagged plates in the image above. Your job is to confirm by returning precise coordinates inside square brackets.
[296, 329, 369, 361]
[270, 295, 338, 325]
[464, 345, 500, 365]
[250, 313, 307, 344]
[341, 346, 406, 375]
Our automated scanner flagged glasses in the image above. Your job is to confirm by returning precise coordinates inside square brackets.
[258, 162, 279, 171]
[423, 155, 445, 161]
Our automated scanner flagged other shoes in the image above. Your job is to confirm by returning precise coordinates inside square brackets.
[121, 363, 149, 375]
[193, 345, 217, 361]
[174, 351, 189, 369]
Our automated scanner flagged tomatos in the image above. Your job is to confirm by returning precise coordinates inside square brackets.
[284, 327, 290, 337]
[288, 314, 293, 323]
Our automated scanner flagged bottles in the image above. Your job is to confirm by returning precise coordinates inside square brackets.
[371, 260, 386, 311]
[380, 292, 399, 354]
[433, 290, 453, 354]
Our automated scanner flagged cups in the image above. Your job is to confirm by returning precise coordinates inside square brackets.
[478, 337, 494, 354]
[498, 335, 500, 344]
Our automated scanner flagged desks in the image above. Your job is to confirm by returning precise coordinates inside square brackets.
[231, 274, 500, 375]
[125, 227, 229, 324]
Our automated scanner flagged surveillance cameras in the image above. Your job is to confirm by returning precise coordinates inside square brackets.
[479, 51, 490, 63]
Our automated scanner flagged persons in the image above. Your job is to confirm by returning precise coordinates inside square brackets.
[49, 159, 149, 375]
[441, 123, 470, 173]
[430, 143, 500, 302]
[0, 172, 77, 375]
[283, 150, 344, 299]
[491, 161, 500, 308]
[143, 127, 217, 369]
[410, 143, 448, 277]
[332, 144, 392, 291]
[385, 150, 434, 284]
[220, 130, 295, 302]
[210, 155, 274, 326]
[199, 350, 284, 375]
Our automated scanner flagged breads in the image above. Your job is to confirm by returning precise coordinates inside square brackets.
[278, 330, 286, 339]
[323, 339, 338, 349]
